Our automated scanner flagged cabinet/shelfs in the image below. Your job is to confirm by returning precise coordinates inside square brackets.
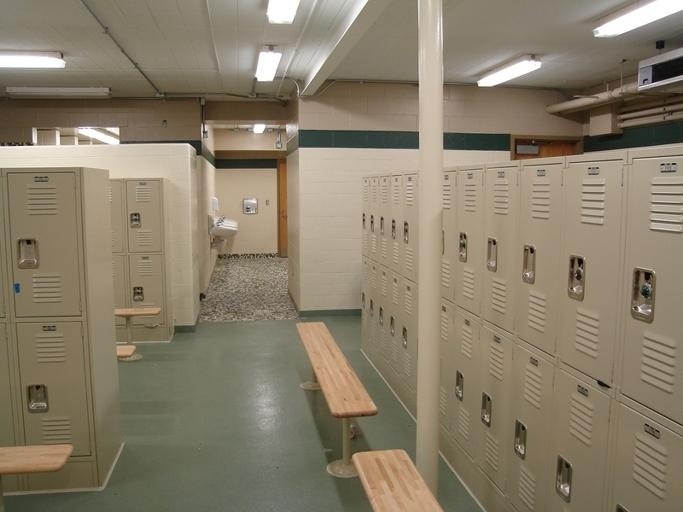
[7, 171, 85, 319]
[130, 254, 165, 329]
[398, 172, 418, 285]
[111, 255, 126, 328]
[552, 160, 624, 390]
[438, 172, 457, 299]
[608, 405, 681, 512]
[436, 299, 454, 429]
[506, 344, 553, 507]
[512, 163, 563, 356]
[614, 154, 683, 424]
[549, 368, 610, 512]
[451, 169, 482, 315]
[359, 174, 390, 268]
[447, 311, 479, 453]
[109, 182, 123, 252]
[125, 181, 162, 252]
[15, 322, 91, 459]
[473, 323, 515, 496]
[480, 167, 520, 336]
[358, 257, 416, 393]
[389, 174, 401, 271]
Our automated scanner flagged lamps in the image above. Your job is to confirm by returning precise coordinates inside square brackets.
[475, 0, 683, 89]
[0, 48, 112, 100]
[255, 0, 300, 86]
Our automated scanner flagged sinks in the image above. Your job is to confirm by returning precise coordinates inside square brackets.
[209, 227, 237, 237]
[215, 218, 238, 228]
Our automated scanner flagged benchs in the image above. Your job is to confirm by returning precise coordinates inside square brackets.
[114, 305, 162, 317]
[293, 317, 444, 512]
[0, 442, 71, 474]
[117, 345, 135, 359]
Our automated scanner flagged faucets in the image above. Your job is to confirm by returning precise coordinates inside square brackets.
[219, 217, 224, 221]
[216, 221, 223, 227]
